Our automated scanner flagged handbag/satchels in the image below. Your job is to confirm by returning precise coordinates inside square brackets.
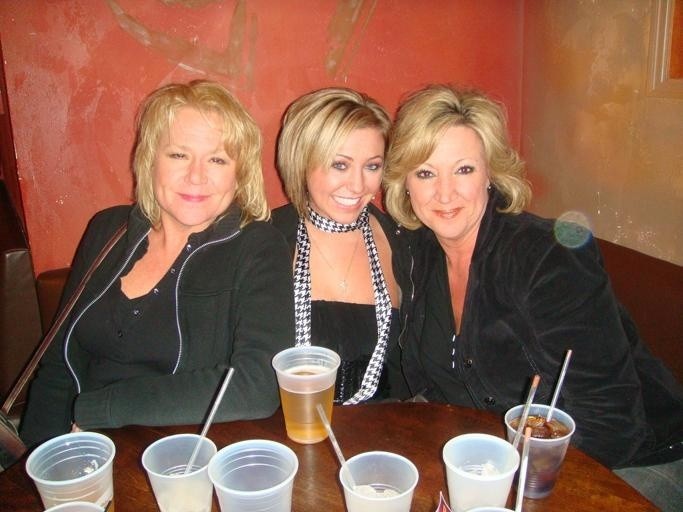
[0, 411, 28, 473]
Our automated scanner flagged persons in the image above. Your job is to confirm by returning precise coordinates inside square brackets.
[380, 82, 683, 512]
[264, 86, 412, 409]
[19, 79, 290, 457]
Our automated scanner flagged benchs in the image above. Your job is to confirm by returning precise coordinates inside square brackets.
[34, 238, 682, 389]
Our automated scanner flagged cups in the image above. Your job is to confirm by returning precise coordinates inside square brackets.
[141, 432, 218, 512]
[272, 346, 340, 444]
[442, 433, 521, 512]
[207, 439, 299, 512]
[504, 405, 576, 499]
[43, 501, 106, 512]
[339, 451, 419, 512]
[26, 431, 116, 512]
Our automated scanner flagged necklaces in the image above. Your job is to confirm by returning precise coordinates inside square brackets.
[304, 228, 361, 296]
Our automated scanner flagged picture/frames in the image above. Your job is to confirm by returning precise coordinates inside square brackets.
[646, 0, 683, 102]
